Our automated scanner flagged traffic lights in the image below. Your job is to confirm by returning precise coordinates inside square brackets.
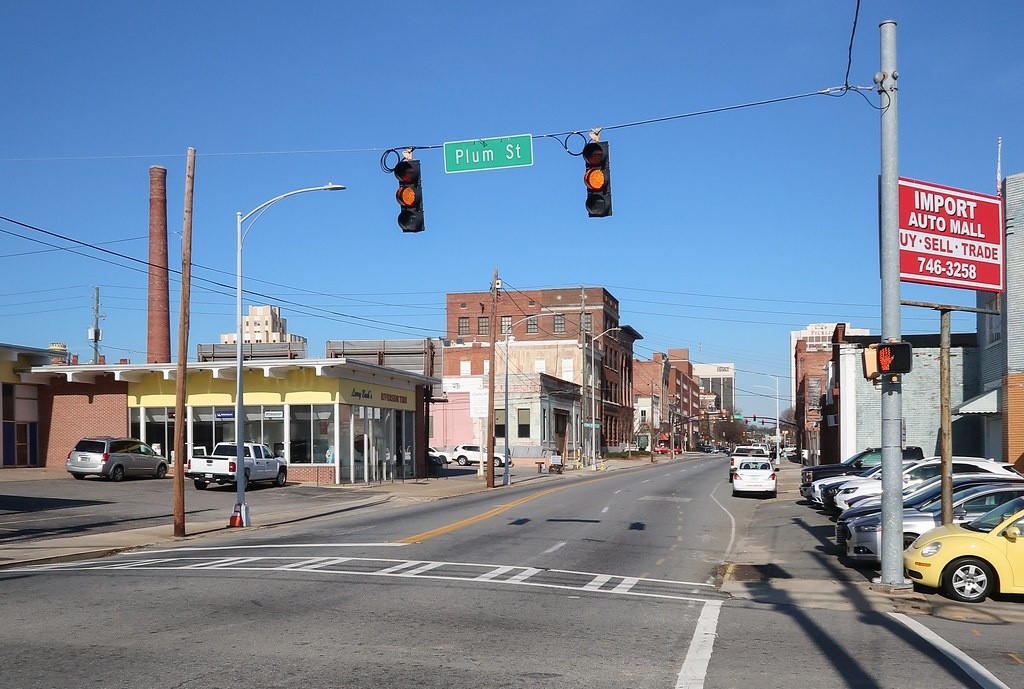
[392, 159, 427, 235]
[754, 414, 756, 421]
[730, 415, 734, 423]
[860, 348, 880, 381]
[762, 419, 764, 425]
[582, 141, 616, 218]
[876, 340, 913, 374]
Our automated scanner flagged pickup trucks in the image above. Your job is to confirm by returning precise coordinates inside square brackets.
[182, 440, 289, 489]
[800, 446, 928, 485]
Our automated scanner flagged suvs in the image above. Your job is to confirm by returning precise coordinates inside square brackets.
[65, 436, 170, 482]
[452, 445, 512, 468]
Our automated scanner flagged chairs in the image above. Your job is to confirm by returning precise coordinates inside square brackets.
[761, 464, 767, 469]
[744, 464, 750, 469]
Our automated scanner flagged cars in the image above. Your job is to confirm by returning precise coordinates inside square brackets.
[731, 458, 781, 498]
[806, 454, 1024, 514]
[726, 443, 776, 483]
[384, 446, 453, 465]
[654, 445, 682, 455]
[845, 484, 1024, 571]
[275, 442, 328, 462]
[700, 445, 714, 452]
[903, 496, 1024, 603]
[835, 474, 1024, 546]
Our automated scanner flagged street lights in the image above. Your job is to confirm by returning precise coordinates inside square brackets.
[228, 182, 346, 528]
[590, 327, 623, 474]
[502, 310, 566, 486]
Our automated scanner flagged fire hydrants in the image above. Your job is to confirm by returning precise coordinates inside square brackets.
[600, 461, 605, 472]
